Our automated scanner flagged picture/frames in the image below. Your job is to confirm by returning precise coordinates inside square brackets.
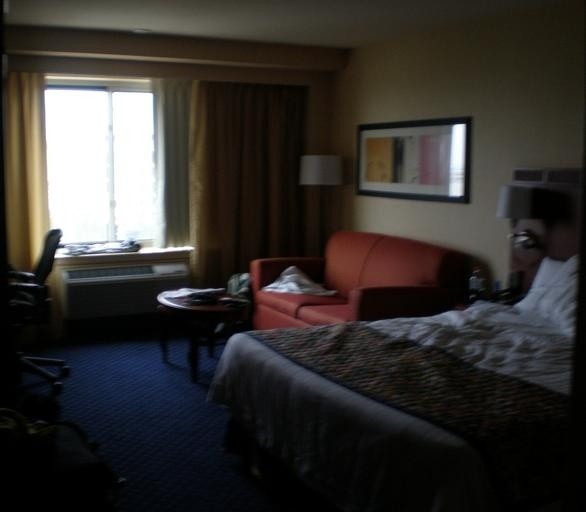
[354, 116, 473, 205]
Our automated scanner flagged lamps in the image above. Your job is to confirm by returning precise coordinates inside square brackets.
[298, 155, 344, 257]
[495, 185, 540, 251]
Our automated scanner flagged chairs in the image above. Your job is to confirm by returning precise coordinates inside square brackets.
[6, 229, 70, 395]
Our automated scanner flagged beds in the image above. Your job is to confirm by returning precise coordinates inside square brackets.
[220, 302, 579, 511]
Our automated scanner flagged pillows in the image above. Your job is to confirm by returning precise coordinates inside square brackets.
[511, 253, 582, 339]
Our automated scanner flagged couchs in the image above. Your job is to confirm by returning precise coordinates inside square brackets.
[246, 230, 466, 331]
